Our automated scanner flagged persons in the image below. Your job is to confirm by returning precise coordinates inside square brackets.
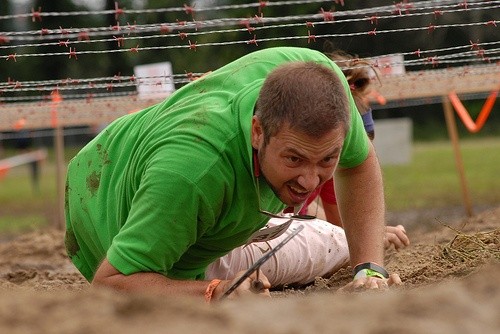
[63, 47, 410, 303]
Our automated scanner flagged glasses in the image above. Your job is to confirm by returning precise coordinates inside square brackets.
[254, 156, 319, 219]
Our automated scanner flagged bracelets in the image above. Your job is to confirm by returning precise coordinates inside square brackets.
[205, 278, 222, 304]
[353, 262, 390, 280]
